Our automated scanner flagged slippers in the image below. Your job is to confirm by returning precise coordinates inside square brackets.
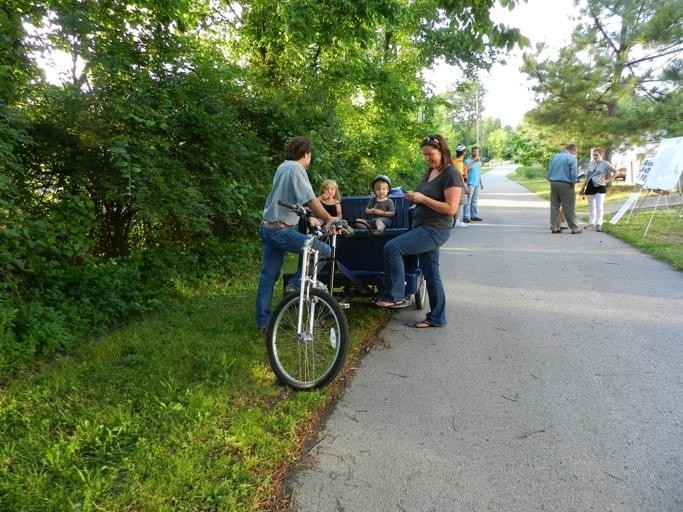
[415, 321, 445, 328]
[374, 297, 405, 308]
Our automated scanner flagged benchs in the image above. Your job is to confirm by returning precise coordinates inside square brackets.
[339, 192, 411, 239]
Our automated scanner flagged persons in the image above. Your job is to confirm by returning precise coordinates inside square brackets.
[357, 173, 397, 232]
[550, 203, 568, 229]
[579, 147, 618, 232]
[307, 179, 344, 233]
[373, 133, 464, 329]
[547, 143, 584, 234]
[451, 144, 469, 219]
[460, 146, 484, 223]
[250, 135, 343, 333]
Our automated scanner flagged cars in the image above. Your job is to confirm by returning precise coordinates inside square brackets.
[482, 156, 489, 162]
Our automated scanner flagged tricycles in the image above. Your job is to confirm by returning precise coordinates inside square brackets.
[262, 184, 428, 392]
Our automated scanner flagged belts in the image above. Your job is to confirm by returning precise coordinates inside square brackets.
[261, 220, 289, 229]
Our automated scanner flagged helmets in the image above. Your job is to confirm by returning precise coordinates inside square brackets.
[370, 174, 391, 197]
[455, 144, 466, 152]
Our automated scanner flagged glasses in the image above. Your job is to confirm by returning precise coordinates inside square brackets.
[422, 136, 439, 144]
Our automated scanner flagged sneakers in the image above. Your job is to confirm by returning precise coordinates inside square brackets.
[551, 226, 569, 234]
[462, 216, 482, 223]
[584, 224, 603, 232]
[572, 228, 584, 234]
[284, 282, 300, 293]
